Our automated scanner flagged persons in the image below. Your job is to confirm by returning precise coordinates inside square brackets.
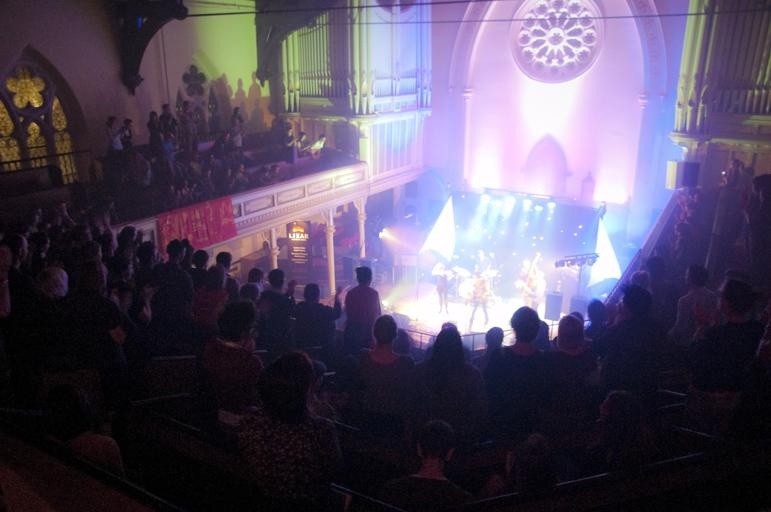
[2, 98, 771, 512]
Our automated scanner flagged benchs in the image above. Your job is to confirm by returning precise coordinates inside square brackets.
[144, 128, 310, 184]
